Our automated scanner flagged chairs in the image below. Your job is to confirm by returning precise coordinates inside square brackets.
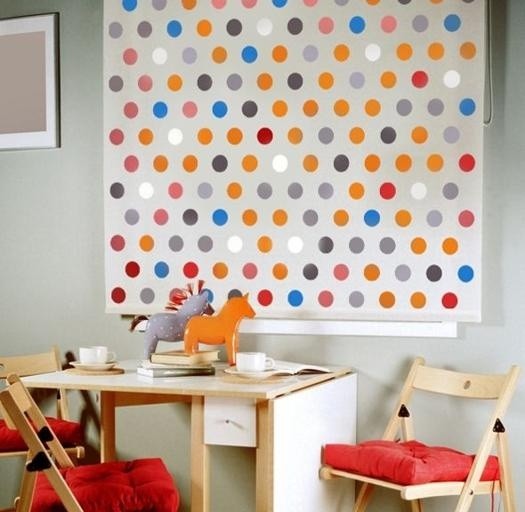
[0, 371, 182, 512]
[0, 342, 87, 464]
[320, 357, 520, 512]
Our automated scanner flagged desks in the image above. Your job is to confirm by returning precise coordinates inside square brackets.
[4, 357, 359, 512]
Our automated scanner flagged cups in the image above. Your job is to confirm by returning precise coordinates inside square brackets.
[235, 351, 274, 371]
[77, 345, 116, 365]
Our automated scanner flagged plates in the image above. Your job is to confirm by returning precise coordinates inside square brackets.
[68, 361, 118, 372]
[224, 365, 280, 381]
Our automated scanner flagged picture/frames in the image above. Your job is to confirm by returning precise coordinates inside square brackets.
[0, 11, 62, 153]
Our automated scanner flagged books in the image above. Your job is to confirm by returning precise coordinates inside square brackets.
[137, 367, 217, 377]
[142, 361, 207, 370]
[224, 359, 334, 381]
[150, 348, 220, 365]
